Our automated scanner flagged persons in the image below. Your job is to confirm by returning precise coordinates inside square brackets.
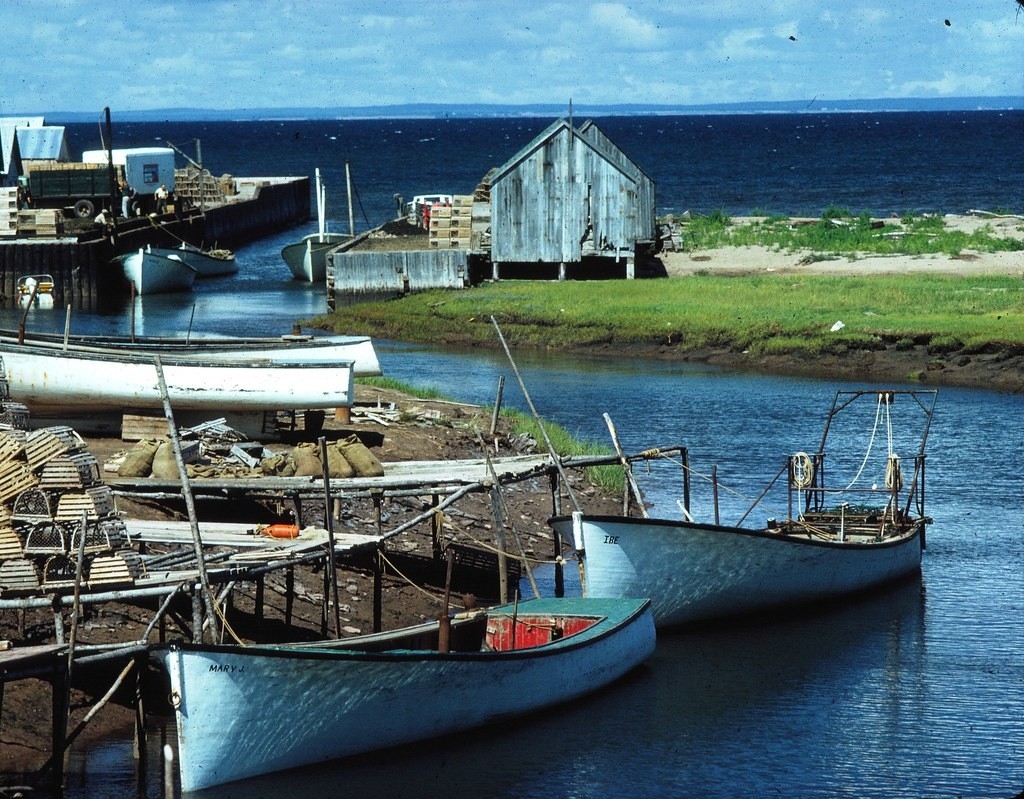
[93, 208, 108, 238]
[119, 180, 130, 222]
[154, 184, 168, 216]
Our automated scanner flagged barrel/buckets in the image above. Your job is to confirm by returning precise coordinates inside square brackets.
[335, 407, 350, 424]
[293, 325, 301, 335]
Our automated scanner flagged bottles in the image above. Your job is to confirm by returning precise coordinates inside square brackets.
[261, 525, 299, 537]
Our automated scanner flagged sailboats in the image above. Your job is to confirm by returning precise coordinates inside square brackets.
[301, 156, 371, 244]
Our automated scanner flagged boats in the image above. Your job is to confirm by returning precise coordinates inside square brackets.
[123, 247, 200, 295]
[16, 273, 55, 311]
[145, 236, 241, 276]
[0, 324, 385, 412]
[281, 166, 338, 282]
[544, 381, 938, 635]
[137, 354, 663, 799]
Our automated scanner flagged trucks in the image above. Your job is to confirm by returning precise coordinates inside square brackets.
[3, 163, 113, 221]
